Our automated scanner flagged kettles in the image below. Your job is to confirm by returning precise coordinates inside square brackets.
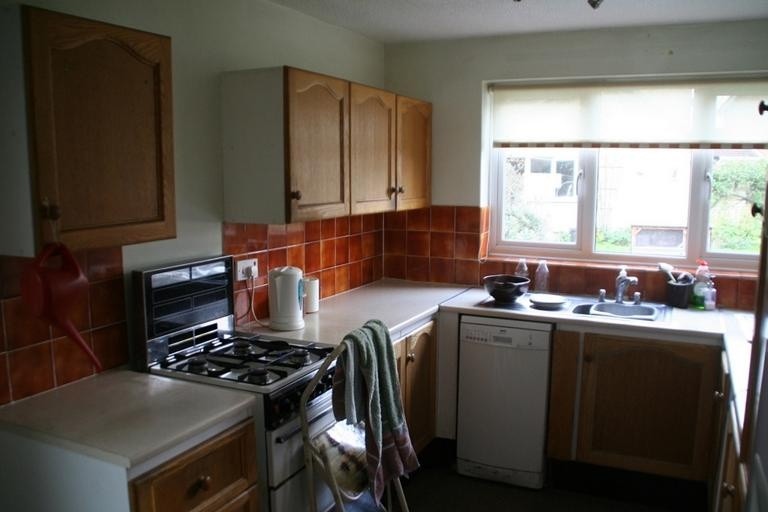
[267, 265, 305, 331]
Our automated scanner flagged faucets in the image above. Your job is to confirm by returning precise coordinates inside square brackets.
[614, 269, 638, 302]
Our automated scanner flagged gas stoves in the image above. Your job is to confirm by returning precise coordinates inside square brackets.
[150, 331, 338, 393]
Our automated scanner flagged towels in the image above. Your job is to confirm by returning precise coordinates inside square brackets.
[331, 317, 420, 508]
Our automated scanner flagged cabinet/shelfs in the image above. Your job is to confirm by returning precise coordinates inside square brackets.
[575, 331, 722, 487]
[1, 2, 177, 258]
[349, 81, 433, 217]
[716, 400, 746, 511]
[389, 320, 437, 455]
[709, 351, 729, 511]
[222, 65, 350, 224]
[0, 365, 264, 512]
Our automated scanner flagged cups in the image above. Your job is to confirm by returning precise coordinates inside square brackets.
[304, 278, 320, 313]
[666, 279, 690, 308]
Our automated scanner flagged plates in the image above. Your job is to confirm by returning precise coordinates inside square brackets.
[530, 293, 566, 308]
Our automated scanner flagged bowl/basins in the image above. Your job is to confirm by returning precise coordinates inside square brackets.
[482, 274, 531, 305]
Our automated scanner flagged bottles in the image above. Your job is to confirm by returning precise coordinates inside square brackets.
[534, 258, 550, 293]
[515, 257, 529, 277]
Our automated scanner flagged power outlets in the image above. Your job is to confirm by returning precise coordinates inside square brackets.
[236, 258, 258, 282]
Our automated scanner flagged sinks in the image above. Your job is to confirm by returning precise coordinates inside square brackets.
[572, 299, 672, 321]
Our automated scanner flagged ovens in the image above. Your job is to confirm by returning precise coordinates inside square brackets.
[266, 386, 336, 512]
